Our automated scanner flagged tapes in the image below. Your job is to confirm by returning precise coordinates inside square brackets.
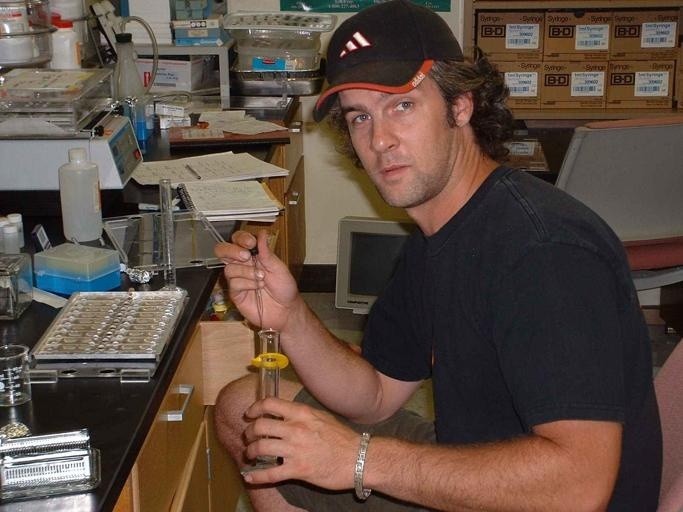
[213, 290, 231, 312]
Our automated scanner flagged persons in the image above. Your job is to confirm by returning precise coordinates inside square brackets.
[213, 0, 663, 509]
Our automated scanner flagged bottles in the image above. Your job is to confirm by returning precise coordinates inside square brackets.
[0, 213, 25, 255]
[113, 33, 145, 99]
[58, 147, 104, 243]
[50, 21, 82, 69]
[241, 330, 285, 479]
[158, 180, 189, 296]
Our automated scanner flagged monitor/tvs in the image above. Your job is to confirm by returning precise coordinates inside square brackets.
[334, 215, 416, 315]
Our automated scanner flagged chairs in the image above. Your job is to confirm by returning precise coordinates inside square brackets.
[652, 336, 683, 512]
[555, 115, 683, 290]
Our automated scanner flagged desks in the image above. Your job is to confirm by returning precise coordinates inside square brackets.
[0, 88, 307, 508]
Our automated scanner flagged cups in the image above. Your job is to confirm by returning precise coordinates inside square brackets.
[0, 345, 32, 409]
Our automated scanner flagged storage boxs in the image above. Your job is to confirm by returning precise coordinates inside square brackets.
[476, 5, 683, 112]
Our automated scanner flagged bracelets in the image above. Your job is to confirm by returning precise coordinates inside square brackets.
[351, 430, 373, 501]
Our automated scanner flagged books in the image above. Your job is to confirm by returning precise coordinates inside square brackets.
[128, 108, 292, 224]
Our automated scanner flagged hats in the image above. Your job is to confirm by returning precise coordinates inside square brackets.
[313, 0, 464, 122]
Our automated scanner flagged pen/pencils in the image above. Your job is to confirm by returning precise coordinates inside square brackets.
[186, 163, 202, 180]
[119, 203, 186, 211]
